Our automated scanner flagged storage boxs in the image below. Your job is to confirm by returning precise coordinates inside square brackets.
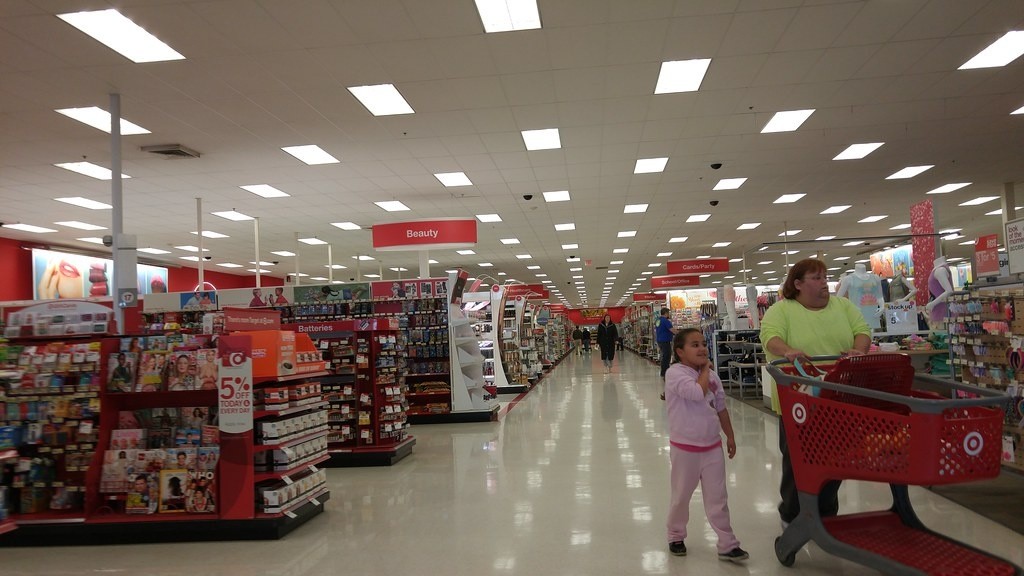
[252, 382, 328, 513]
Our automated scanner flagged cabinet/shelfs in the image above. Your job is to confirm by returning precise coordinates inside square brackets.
[314, 301, 575, 548]
[0, 337, 221, 523]
[870, 332, 965, 397]
[620, 300, 764, 402]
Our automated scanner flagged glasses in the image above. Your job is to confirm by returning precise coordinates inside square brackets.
[801, 275, 829, 281]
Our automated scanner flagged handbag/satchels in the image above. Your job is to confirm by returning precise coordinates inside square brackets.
[795, 355, 828, 396]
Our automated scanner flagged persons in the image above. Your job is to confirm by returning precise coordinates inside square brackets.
[616, 322, 624, 351]
[889, 270, 918, 305]
[112, 406, 219, 513]
[320, 285, 368, 300]
[152, 339, 161, 350]
[436, 282, 447, 293]
[837, 264, 884, 328]
[664, 328, 749, 561]
[391, 282, 400, 298]
[596, 314, 619, 373]
[582, 328, 590, 352]
[572, 325, 583, 355]
[269, 287, 289, 306]
[249, 288, 268, 307]
[127, 338, 141, 352]
[199, 350, 218, 389]
[759, 259, 871, 532]
[656, 308, 678, 382]
[926, 256, 953, 331]
[186, 292, 211, 305]
[168, 354, 195, 390]
[142, 354, 170, 392]
[111, 353, 132, 392]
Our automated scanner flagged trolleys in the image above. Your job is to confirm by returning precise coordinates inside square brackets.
[765, 355, 1024, 576]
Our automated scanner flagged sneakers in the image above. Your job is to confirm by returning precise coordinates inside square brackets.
[669, 541, 686, 556]
[718, 547, 748, 561]
